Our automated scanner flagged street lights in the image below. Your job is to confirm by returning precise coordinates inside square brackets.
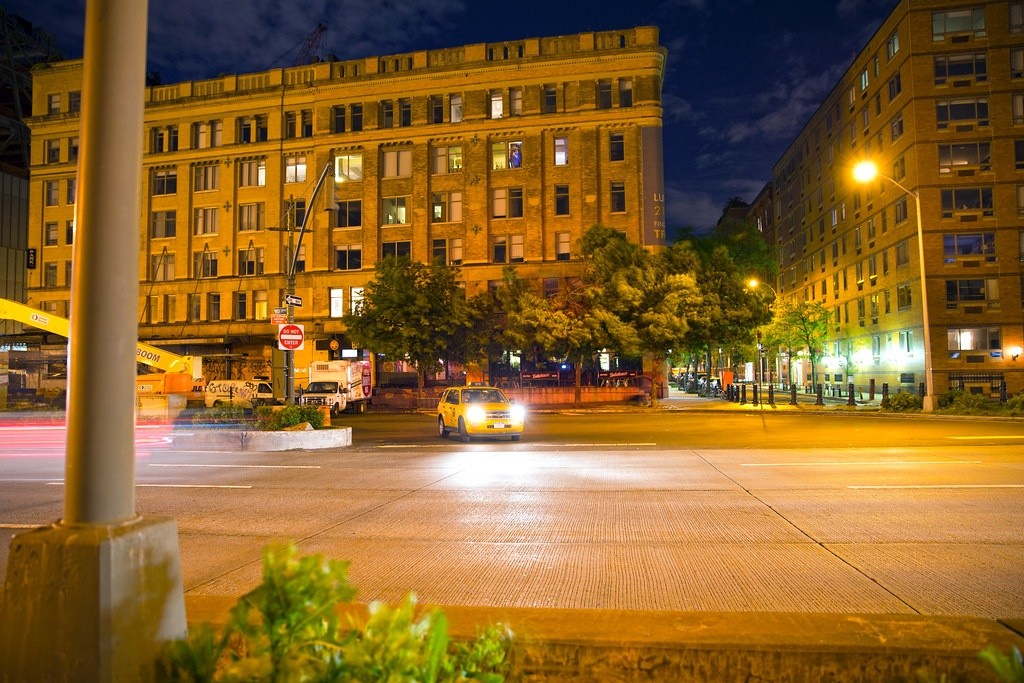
[748, 278, 784, 390]
[852, 161, 939, 415]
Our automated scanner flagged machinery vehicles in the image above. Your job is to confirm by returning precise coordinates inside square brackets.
[0, 297, 208, 421]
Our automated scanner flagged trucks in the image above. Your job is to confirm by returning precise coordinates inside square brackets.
[297, 359, 372, 419]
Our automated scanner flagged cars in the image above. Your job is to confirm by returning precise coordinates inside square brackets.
[436, 381, 524, 442]
[677, 371, 740, 398]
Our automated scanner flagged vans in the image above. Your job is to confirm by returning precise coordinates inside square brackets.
[204, 380, 286, 409]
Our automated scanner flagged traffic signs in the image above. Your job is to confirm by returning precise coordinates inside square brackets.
[286, 294, 302, 307]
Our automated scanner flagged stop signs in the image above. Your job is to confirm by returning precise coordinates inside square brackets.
[279, 325, 304, 349]
